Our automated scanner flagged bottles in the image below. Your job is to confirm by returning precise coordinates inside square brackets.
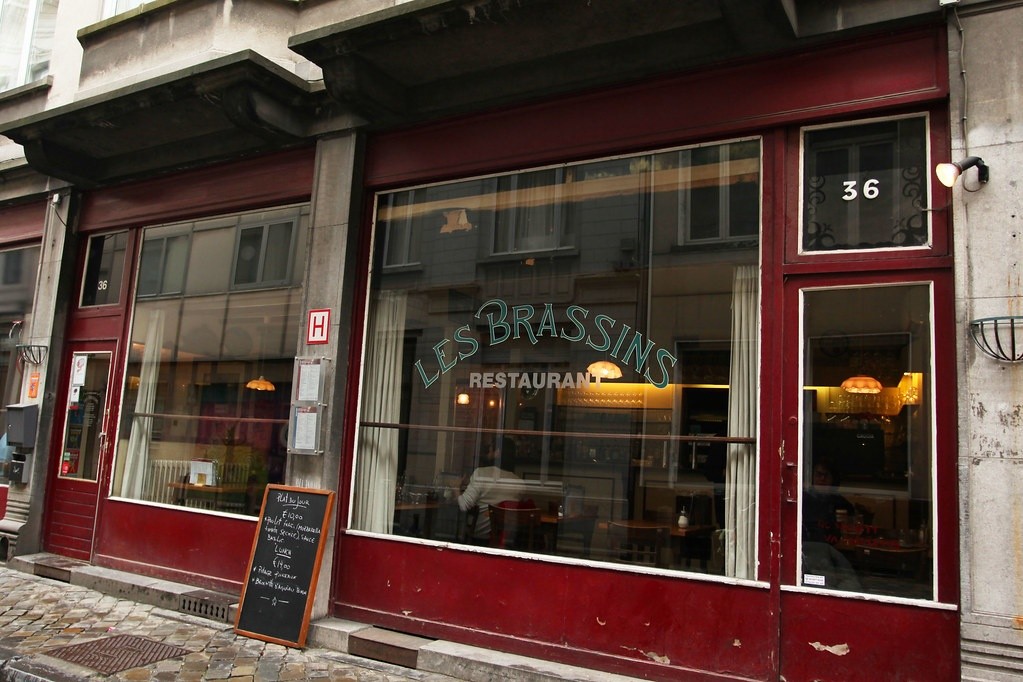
[918, 524, 927, 543]
[678, 510, 690, 528]
[557, 505, 564, 517]
[898, 529, 906, 546]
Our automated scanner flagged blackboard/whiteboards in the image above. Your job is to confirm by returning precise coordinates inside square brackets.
[231, 482, 336, 651]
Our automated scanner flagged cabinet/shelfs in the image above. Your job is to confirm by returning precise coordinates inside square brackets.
[679, 387, 730, 486]
[550, 382, 675, 470]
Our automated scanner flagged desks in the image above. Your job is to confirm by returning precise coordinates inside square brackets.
[833, 535, 933, 558]
[395, 501, 460, 542]
[599, 520, 716, 573]
[167, 483, 265, 517]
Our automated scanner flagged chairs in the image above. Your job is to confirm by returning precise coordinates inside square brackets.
[856, 548, 929, 597]
[489, 499, 599, 559]
[607, 495, 714, 573]
[247, 483, 285, 516]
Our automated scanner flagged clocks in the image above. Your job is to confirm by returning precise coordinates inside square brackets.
[819, 330, 847, 357]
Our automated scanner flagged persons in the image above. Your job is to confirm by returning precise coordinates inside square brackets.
[449, 437, 527, 541]
[802, 456, 862, 591]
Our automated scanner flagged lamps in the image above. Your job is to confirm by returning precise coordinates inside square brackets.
[937, 157, 990, 187]
[840, 337, 884, 393]
[246, 317, 275, 391]
[586, 349, 623, 379]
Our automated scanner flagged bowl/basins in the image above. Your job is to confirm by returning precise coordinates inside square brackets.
[406, 492, 429, 503]
